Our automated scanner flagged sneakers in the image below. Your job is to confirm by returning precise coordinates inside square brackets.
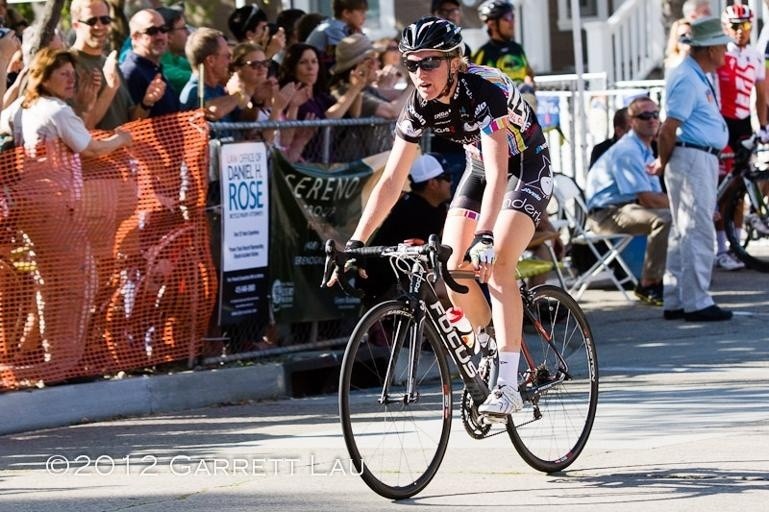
[664, 309, 686, 321]
[475, 354, 500, 392]
[714, 250, 745, 273]
[478, 385, 524, 418]
[684, 305, 733, 323]
[634, 282, 666, 306]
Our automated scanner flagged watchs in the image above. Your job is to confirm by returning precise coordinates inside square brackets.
[140, 102, 159, 114]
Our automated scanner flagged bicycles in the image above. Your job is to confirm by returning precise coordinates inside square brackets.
[717, 123, 768, 272]
[319, 233, 601, 499]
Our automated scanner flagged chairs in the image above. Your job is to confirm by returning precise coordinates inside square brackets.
[483, 253, 562, 317]
[547, 173, 641, 306]
[526, 227, 576, 298]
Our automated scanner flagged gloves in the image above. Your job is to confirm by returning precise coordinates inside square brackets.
[335, 238, 365, 271]
[758, 125, 768, 148]
[461, 232, 498, 268]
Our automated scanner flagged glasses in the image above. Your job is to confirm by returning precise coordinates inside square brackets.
[630, 111, 660, 119]
[170, 22, 190, 34]
[211, 51, 234, 60]
[137, 25, 170, 37]
[246, 59, 272, 69]
[399, 56, 458, 73]
[497, 12, 515, 24]
[432, 8, 461, 19]
[77, 16, 113, 27]
[385, 44, 398, 54]
[729, 23, 752, 34]
[242, 1, 260, 30]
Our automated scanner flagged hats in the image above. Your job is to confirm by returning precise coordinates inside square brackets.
[408, 149, 461, 189]
[330, 35, 384, 77]
[676, 15, 734, 49]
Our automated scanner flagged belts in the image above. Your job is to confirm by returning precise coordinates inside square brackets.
[586, 205, 602, 218]
[676, 141, 721, 158]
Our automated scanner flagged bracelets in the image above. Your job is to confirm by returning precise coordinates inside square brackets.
[237, 89, 247, 105]
[250, 96, 265, 107]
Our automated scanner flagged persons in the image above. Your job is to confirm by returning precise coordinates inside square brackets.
[227, 5, 287, 59]
[0, 28, 20, 94]
[586, 93, 671, 306]
[326, 16, 554, 416]
[589, 108, 635, 155]
[715, 4, 768, 273]
[377, 41, 401, 67]
[682, 1, 711, 22]
[431, 1, 462, 27]
[653, 18, 734, 321]
[373, 51, 403, 90]
[358, 152, 453, 308]
[272, 9, 305, 59]
[1, 48, 134, 387]
[264, 61, 321, 163]
[328, 32, 413, 120]
[470, 1, 535, 92]
[282, 44, 368, 164]
[61, 1, 167, 268]
[518, 204, 566, 321]
[121, 9, 175, 119]
[375, 39, 401, 67]
[305, 1, 369, 56]
[226, 43, 311, 147]
[179, 28, 279, 121]
[156, 7, 193, 89]
[296, 13, 327, 41]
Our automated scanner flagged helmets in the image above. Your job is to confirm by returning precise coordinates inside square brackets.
[720, 5, 756, 30]
[395, 15, 464, 60]
[476, 0, 515, 21]
